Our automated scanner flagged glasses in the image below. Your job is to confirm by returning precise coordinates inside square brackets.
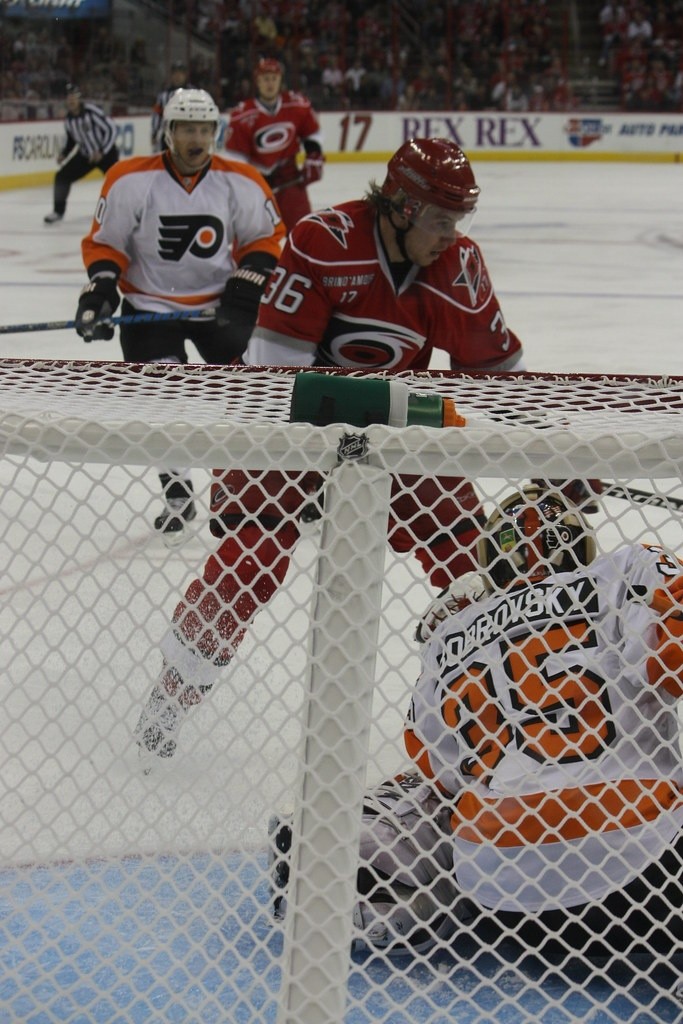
[169, 64, 188, 74]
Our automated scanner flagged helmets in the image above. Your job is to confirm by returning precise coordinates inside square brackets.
[384, 137, 476, 240]
[478, 488, 595, 592]
[251, 59, 285, 83]
[163, 88, 222, 143]
[61, 84, 80, 98]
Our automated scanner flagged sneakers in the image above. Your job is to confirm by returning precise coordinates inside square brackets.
[44, 213, 63, 222]
[270, 766, 455, 951]
[138, 657, 203, 760]
[155, 495, 197, 532]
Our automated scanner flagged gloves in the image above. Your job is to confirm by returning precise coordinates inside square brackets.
[220, 264, 270, 323]
[302, 151, 324, 186]
[75, 275, 120, 342]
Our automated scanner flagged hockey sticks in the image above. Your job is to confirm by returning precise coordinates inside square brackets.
[2, 302, 223, 338]
[272, 172, 304, 197]
[599, 482, 682, 512]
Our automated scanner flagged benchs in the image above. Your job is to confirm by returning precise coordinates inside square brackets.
[566, 74, 625, 111]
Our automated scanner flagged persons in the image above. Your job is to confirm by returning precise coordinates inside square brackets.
[218, 51, 326, 238]
[44, 85, 122, 228]
[267, 483, 682, 977]
[136, 140, 527, 772]
[0, 0, 682, 122]
[73, 88, 290, 550]
